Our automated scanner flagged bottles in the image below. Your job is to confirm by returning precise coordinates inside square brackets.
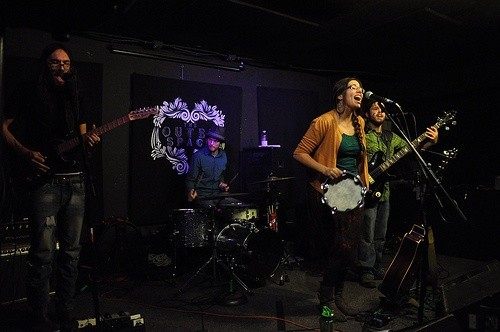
[319, 306, 335, 332]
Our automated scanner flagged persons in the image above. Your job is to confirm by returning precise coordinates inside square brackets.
[184, 125, 241, 207]
[356, 99, 438, 286]
[0, 44, 100, 332]
[294, 76, 375, 322]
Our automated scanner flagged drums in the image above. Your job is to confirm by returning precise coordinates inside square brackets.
[322, 171, 365, 211]
[219, 203, 260, 225]
[168, 207, 214, 248]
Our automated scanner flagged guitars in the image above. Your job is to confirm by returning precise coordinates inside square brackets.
[377, 223, 427, 300]
[22, 104, 157, 180]
[364, 109, 458, 210]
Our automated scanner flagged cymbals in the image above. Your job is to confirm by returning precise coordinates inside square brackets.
[320, 169, 368, 215]
[253, 177, 297, 184]
[199, 191, 249, 199]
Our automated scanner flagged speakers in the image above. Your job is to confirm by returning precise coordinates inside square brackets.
[0, 241, 59, 307]
[409, 266, 500, 332]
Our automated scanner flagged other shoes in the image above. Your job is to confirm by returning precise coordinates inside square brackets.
[361, 272, 376, 287]
[375, 268, 384, 279]
[336, 296, 359, 316]
[320, 297, 347, 322]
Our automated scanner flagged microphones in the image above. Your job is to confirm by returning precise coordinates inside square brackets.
[52, 70, 74, 80]
[385, 113, 409, 119]
[365, 91, 396, 104]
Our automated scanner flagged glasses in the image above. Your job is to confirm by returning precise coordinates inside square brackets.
[346, 84, 365, 93]
[47, 62, 71, 71]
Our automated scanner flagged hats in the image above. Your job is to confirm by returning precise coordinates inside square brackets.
[204, 126, 226, 141]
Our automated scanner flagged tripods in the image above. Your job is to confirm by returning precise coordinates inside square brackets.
[176, 198, 256, 297]
